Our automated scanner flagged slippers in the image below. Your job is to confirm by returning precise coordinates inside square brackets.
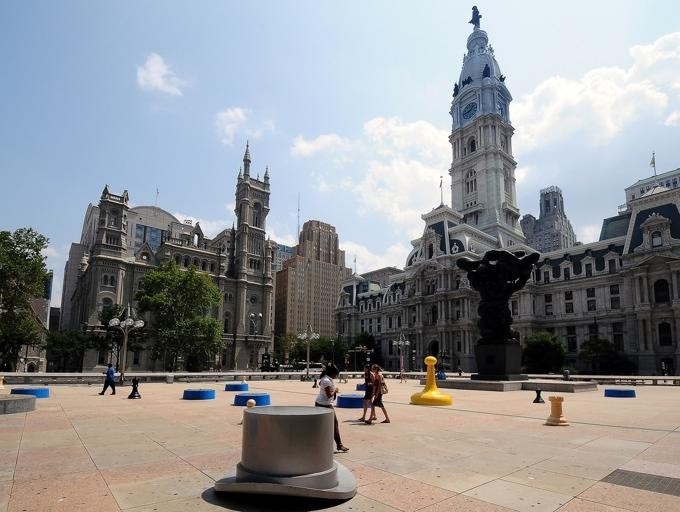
[358, 418, 366, 421]
[381, 420, 390, 423]
[372, 418, 377, 420]
[364, 420, 371, 424]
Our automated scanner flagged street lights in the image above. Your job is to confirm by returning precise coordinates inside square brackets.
[247, 313, 262, 371]
[295, 325, 320, 381]
[329, 339, 335, 364]
[393, 332, 410, 373]
[109, 302, 144, 384]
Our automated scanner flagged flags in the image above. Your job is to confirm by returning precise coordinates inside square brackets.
[650, 156, 655, 167]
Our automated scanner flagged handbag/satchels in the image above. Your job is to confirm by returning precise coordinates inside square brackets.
[380, 382, 388, 395]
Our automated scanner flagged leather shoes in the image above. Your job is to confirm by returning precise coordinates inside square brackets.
[336, 444, 350, 452]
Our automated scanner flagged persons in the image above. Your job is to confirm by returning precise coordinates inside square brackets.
[271, 358, 280, 372]
[314, 362, 350, 453]
[97, 363, 116, 395]
[366, 364, 391, 424]
[399, 367, 407, 383]
[358, 363, 377, 421]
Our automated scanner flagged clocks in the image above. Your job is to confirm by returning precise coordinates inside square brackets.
[463, 102, 477, 119]
